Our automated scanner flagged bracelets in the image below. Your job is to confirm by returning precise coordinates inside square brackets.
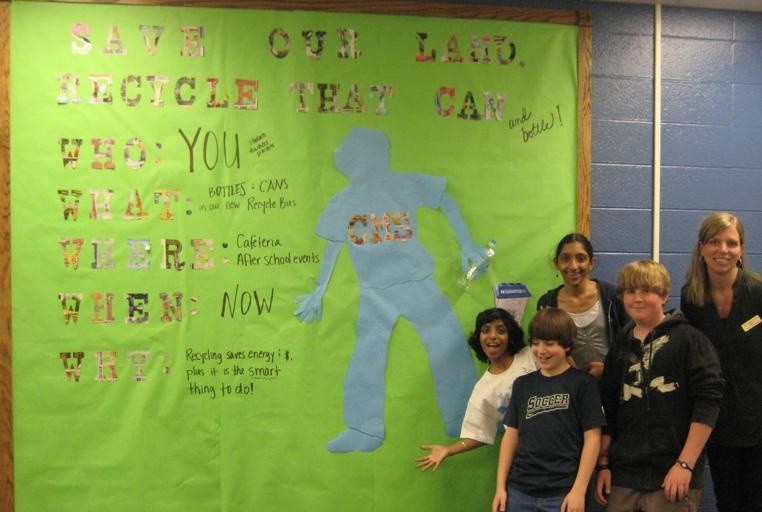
[595, 464, 609, 471]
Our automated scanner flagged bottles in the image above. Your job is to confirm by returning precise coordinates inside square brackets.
[456, 239, 497, 290]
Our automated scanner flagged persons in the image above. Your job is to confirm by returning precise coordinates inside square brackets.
[415, 307, 527, 472]
[536, 232, 630, 512]
[493, 308, 601, 512]
[593, 259, 725, 512]
[677, 212, 762, 512]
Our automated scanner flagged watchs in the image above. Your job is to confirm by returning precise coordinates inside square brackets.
[677, 460, 693, 472]
